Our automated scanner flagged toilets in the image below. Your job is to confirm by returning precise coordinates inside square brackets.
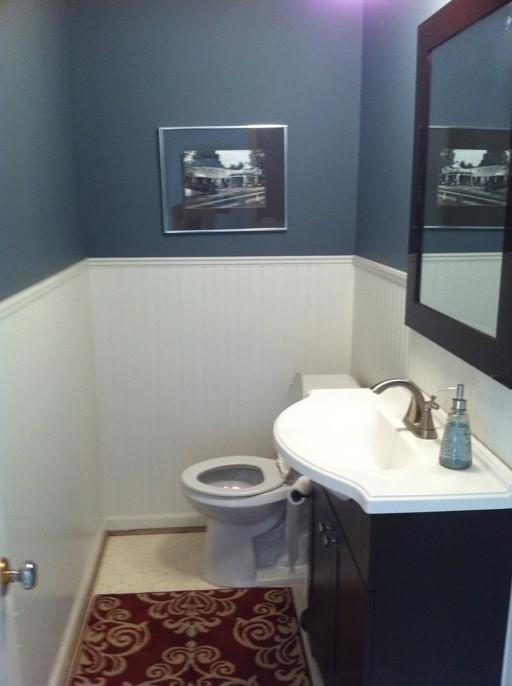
[181, 371, 361, 586]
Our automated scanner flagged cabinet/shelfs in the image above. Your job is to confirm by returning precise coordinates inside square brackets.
[311, 480, 375, 686]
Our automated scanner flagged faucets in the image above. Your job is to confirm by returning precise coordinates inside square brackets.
[369, 380, 441, 443]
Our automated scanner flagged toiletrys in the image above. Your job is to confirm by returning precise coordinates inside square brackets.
[438, 384, 473, 472]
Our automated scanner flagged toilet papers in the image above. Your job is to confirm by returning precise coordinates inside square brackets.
[285, 475, 311, 576]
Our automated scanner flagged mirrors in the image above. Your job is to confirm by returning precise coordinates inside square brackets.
[404, 0, 512, 391]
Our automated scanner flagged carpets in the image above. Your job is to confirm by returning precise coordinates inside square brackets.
[66, 586, 314, 685]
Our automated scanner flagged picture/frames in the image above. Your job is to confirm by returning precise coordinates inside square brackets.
[158, 124, 289, 234]
[423, 125, 511, 230]
